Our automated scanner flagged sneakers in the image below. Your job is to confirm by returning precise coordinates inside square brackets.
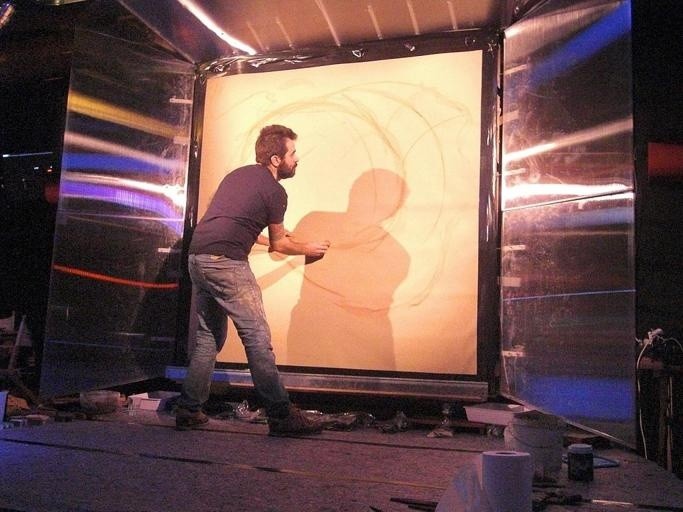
[177, 411, 209, 431]
[269, 407, 322, 437]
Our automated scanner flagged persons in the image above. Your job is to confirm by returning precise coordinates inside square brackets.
[173, 123, 331, 436]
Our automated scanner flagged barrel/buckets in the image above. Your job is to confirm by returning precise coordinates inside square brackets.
[503, 422, 563, 487]
